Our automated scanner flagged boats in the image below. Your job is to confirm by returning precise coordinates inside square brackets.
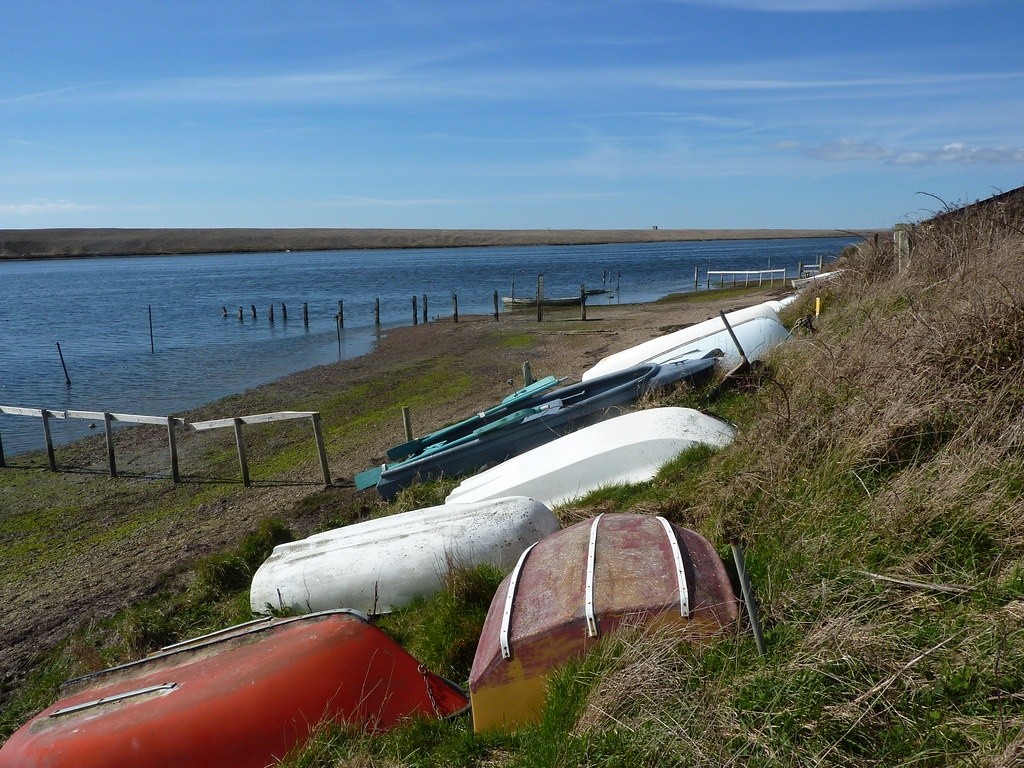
[791, 272, 844, 290]
[0, 604, 468, 768]
[246, 495, 559, 621]
[470, 509, 741, 738]
[502, 294, 587, 312]
[589, 289, 612, 297]
[451, 406, 739, 523]
[378, 294, 799, 500]
